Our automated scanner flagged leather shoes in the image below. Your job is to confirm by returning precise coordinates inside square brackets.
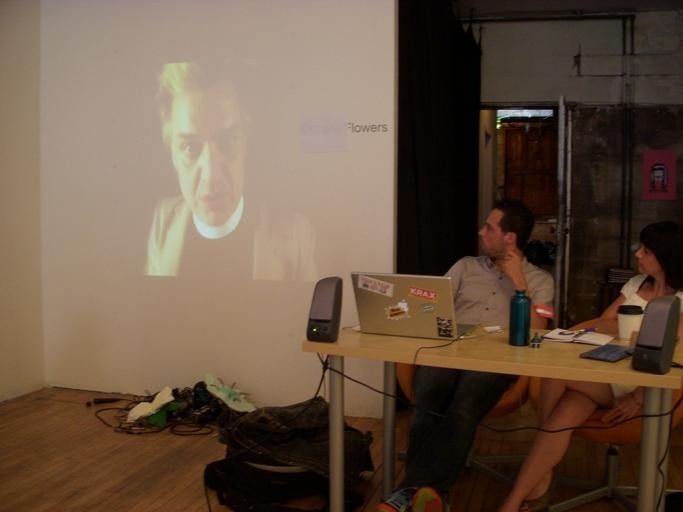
[520, 473, 558, 512]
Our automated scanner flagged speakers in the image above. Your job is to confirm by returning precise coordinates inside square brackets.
[306, 276, 342, 343]
[631, 294, 679, 375]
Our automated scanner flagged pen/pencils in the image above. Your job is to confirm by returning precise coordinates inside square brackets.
[559, 327, 599, 335]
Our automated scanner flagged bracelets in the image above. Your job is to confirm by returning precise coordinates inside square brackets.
[630, 392, 646, 409]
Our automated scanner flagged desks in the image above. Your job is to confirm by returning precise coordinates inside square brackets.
[300, 320, 681, 511]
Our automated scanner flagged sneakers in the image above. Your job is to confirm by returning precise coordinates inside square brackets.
[411, 485, 451, 512]
[377, 485, 411, 512]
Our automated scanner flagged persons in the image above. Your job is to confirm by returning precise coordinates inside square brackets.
[372, 196, 556, 511]
[145, 60, 312, 279]
[498, 220, 683, 511]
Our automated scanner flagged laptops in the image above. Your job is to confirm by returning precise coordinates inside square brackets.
[349, 273, 478, 340]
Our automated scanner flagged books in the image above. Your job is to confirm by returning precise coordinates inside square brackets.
[608, 267, 635, 284]
[543, 327, 616, 346]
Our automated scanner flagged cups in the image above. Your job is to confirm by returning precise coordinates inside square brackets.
[616, 306, 643, 340]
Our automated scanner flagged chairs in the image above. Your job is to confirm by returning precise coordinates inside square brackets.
[395, 355, 532, 467]
[527, 315, 681, 512]
